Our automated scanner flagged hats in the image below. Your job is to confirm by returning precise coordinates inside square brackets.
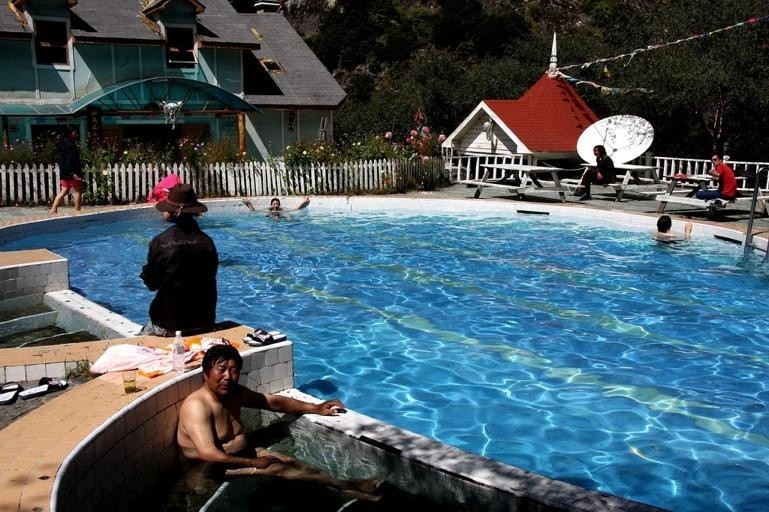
[156, 184, 207, 214]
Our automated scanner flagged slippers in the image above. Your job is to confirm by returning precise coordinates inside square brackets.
[19, 377, 69, 403]
[243, 328, 287, 347]
[0, 381, 18, 405]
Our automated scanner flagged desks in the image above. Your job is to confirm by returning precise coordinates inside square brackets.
[473, 164, 567, 202]
[572, 162, 664, 202]
[655, 174, 747, 219]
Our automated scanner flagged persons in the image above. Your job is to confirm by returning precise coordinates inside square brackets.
[573, 144, 617, 201]
[135, 180, 220, 337]
[656, 213, 693, 241]
[696, 150, 737, 200]
[48, 124, 86, 214]
[242, 195, 310, 213]
[177, 343, 382, 502]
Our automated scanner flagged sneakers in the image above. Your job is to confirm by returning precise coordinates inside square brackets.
[574, 188, 592, 200]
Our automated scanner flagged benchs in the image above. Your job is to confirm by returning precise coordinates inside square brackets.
[453, 174, 670, 204]
[654, 185, 769, 223]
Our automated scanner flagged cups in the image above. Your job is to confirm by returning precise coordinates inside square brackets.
[122, 369, 137, 394]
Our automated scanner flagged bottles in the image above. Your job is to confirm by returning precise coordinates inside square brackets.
[173, 330, 186, 375]
